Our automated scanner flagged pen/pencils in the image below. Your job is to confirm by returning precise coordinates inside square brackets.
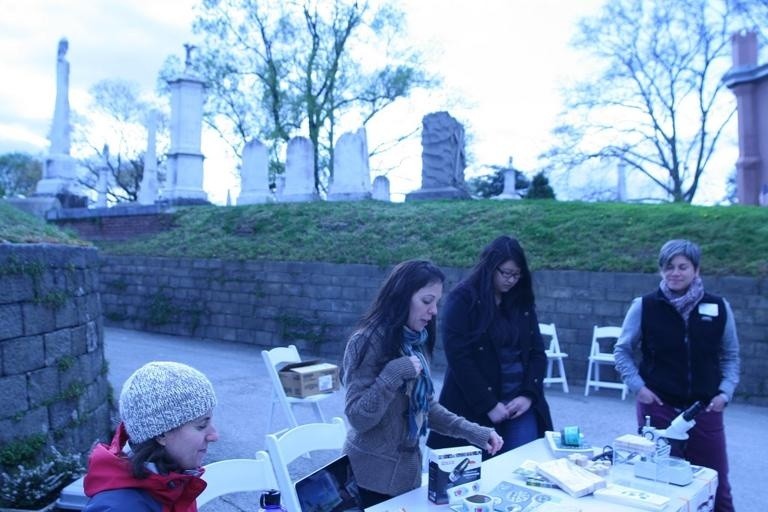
[527, 481, 560, 489]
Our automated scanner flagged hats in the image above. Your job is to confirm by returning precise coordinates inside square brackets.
[118, 359, 217, 445]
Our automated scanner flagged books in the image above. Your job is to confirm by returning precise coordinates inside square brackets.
[486, 431, 706, 512]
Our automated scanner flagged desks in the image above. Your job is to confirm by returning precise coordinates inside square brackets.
[364, 436, 719, 512]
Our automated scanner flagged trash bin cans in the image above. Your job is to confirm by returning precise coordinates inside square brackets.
[53, 476, 91, 512]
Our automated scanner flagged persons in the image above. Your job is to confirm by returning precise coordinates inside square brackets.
[613, 239, 741, 512]
[83, 361, 218, 512]
[426, 236, 553, 461]
[339, 259, 504, 508]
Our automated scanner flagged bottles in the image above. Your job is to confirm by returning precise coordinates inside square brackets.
[258, 490, 285, 512]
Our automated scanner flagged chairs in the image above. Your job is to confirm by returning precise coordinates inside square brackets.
[190, 342, 348, 512]
[539, 320, 629, 399]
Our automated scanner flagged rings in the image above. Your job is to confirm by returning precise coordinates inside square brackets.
[499, 436, 502, 442]
[710, 403, 714, 406]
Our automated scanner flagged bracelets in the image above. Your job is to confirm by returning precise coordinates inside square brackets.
[720, 393, 728, 402]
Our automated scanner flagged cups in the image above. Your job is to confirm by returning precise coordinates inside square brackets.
[462, 494, 494, 512]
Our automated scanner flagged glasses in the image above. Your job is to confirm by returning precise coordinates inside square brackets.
[496, 267, 523, 279]
